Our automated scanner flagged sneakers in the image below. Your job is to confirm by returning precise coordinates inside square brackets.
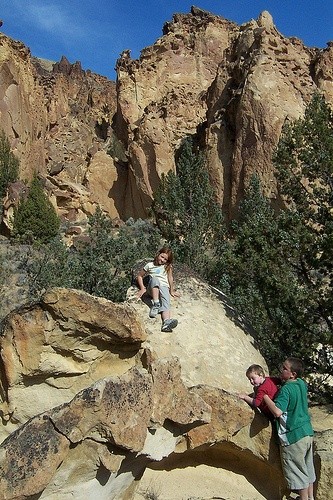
[160, 319, 178, 331]
[150, 299, 161, 317]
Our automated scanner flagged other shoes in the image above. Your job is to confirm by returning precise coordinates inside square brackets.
[296, 496, 314, 500]
